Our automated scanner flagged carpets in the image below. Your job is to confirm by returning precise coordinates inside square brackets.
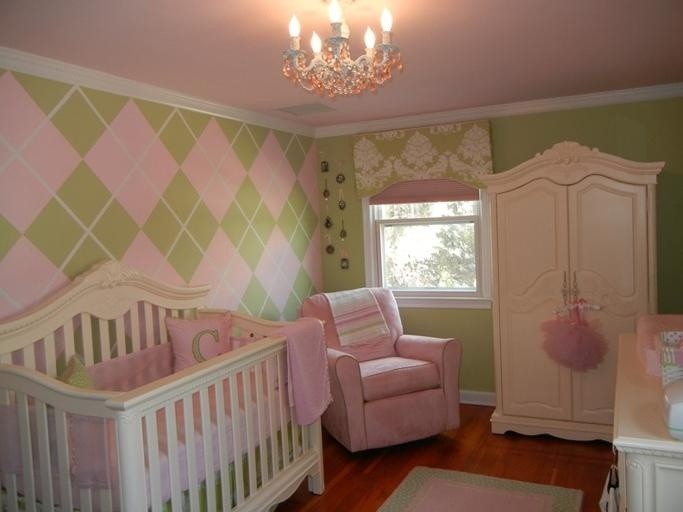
[375, 465, 584, 512]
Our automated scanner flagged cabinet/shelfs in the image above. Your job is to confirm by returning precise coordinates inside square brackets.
[478, 141, 666, 443]
[612, 313, 683, 512]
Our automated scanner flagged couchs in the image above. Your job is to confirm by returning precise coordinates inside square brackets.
[298, 286, 462, 453]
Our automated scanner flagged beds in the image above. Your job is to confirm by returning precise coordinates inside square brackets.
[0, 260, 325, 511]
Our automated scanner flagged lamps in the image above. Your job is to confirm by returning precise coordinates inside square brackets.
[279, 0, 405, 99]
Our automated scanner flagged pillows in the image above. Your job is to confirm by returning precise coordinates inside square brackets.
[165, 311, 232, 373]
[53, 355, 97, 389]
